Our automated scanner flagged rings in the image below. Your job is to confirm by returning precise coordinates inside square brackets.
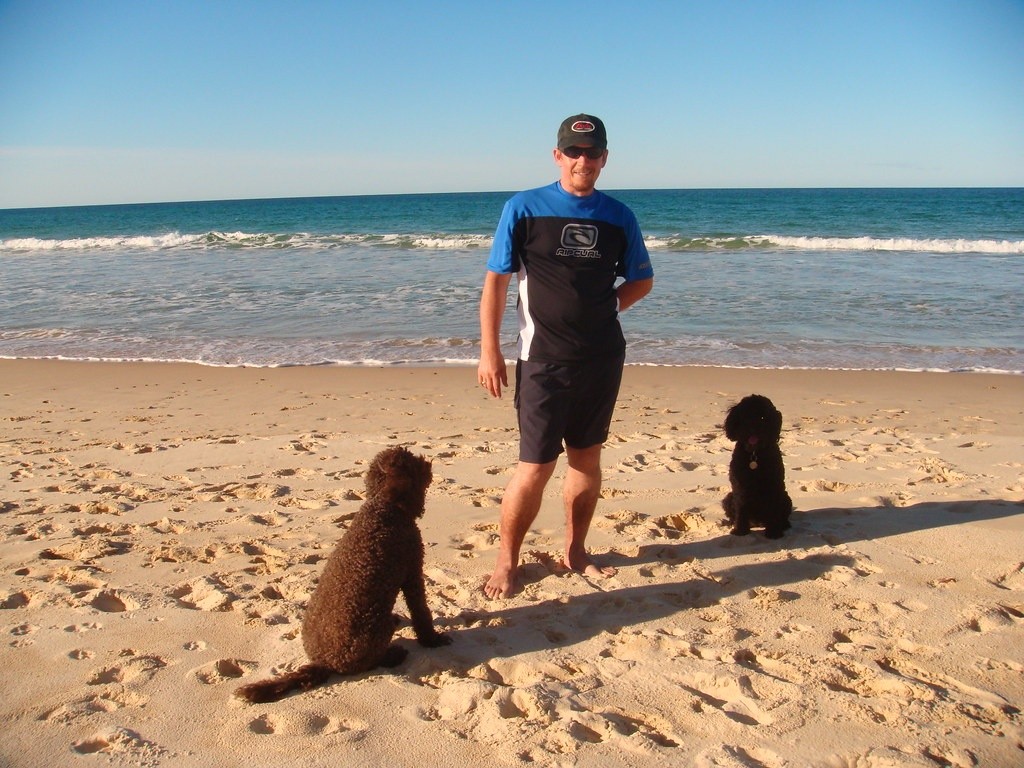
[482, 382, 486, 384]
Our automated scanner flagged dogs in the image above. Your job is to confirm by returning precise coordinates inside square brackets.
[233, 445, 453, 706]
[721, 393, 792, 540]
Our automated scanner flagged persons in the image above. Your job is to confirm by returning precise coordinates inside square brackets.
[477, 113, 653, 600]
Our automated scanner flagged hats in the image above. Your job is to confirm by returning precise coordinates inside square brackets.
[557, 113, 608, 151]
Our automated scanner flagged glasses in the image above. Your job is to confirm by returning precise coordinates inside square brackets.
[561, 146, 606, 159]
